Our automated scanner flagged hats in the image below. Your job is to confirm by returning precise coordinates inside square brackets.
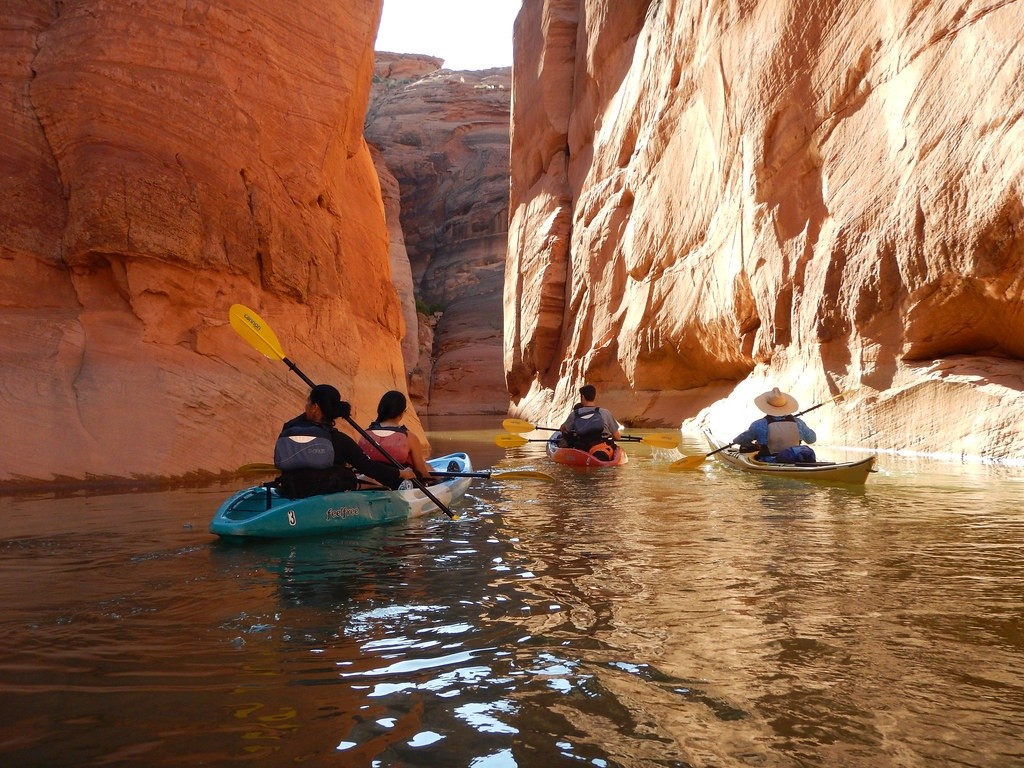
[754, 387, 799, 417]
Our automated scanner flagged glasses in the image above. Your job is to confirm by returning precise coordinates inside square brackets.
[403, 408, 407, 413]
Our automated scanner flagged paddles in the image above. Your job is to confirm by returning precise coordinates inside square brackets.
[230, 301, 461, 520]
[503, 418, 680, 448]
[669, 387, 858, 470]
[494, 433, 678, 448]
[237, 462, 556, 482]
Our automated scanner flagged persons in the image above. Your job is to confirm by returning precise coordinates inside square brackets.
[359, 390, 444, 482]
[274, 383, 417, 499]
[557, 384, 621, 449]
[734, 387, 817, 458]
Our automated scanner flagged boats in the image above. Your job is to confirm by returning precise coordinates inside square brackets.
[545, 428, 629, 468]
[208, 451, 473, 543]
[704, 427, 877, 489]
